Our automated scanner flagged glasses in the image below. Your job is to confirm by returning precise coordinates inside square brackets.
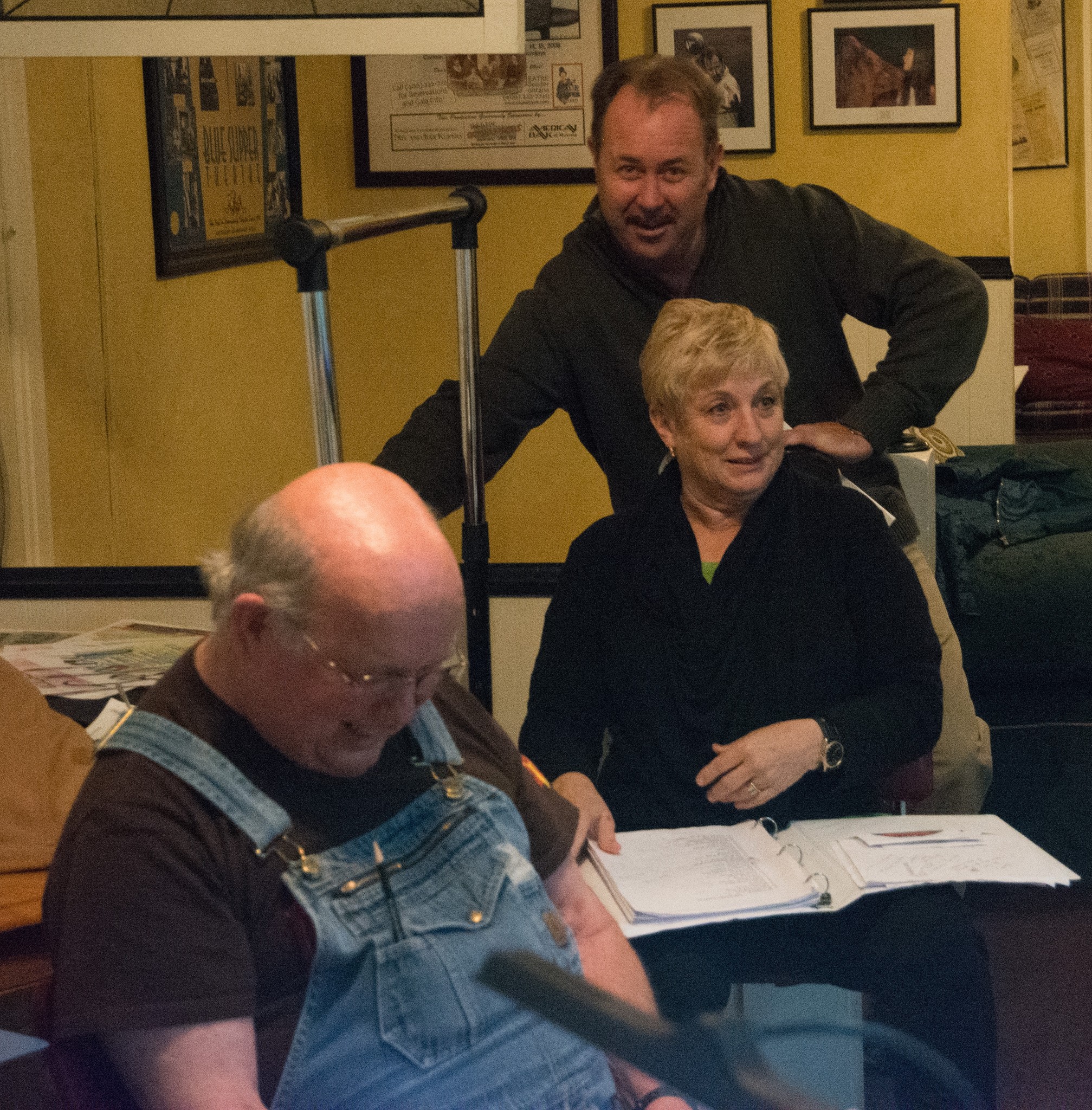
[282, 610, 467, 701]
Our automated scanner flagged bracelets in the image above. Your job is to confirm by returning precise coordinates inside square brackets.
[634, 1084, 690, 1110]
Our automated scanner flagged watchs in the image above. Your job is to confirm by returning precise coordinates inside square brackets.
[811, 714, 844, 772]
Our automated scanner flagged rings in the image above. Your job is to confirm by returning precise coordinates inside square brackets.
[746, 781, 761, 797]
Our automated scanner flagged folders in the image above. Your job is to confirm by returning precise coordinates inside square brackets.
[550, 810, 1081, 945]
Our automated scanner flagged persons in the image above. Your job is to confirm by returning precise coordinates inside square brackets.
[894, 43, 935, 105]
[834, 46, 905, 107]
[691, 47, 742, 128]
[448, 52, 524, 91]
[514, 297, 945, 861]
[38, 460, 693, 1110]
[156, 57, 290, 246]
[372, 51, 994, 819]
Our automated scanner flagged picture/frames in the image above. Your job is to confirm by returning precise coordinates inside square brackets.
[1012, 1, 1069, 171]
[806, 4, 962, 130]
[349, 1, 618, 190]
[651, 0, 778, 156]
[140, 56, 302, 282]
